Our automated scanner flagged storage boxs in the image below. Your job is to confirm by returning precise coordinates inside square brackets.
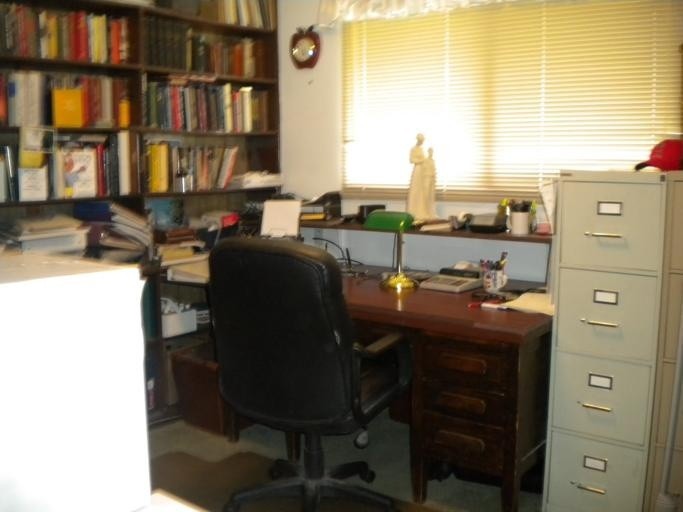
[170, 340, 257, 437]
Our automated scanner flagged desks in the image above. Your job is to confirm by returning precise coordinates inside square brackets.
[149, 218, 553, 511]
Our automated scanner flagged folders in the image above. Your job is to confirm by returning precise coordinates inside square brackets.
[83, 222, 145, 251]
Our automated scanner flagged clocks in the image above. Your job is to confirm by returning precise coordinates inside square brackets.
[289, 26, 322, 70]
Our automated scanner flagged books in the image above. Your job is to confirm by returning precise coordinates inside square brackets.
[1, 1, 284, 282]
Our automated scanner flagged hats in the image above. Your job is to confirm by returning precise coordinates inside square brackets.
[636, 140, 683, 170]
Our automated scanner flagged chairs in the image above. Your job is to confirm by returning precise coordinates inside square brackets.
[204, 235, 420, 512]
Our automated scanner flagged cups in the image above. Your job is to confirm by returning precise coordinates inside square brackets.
[483, 271, 507, 294]
[510, 212, 529, 235]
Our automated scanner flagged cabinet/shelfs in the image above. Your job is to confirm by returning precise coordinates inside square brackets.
[534, 168, 683, 512]
[2, 0, 287, 432]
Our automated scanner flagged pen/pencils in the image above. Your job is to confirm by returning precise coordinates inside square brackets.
[481, 252, 507, 271]
[509, 199, 532, 212]
[465, 300, 502, 307]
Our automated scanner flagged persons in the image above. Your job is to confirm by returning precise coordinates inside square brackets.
[408, 134, 436, 220]
